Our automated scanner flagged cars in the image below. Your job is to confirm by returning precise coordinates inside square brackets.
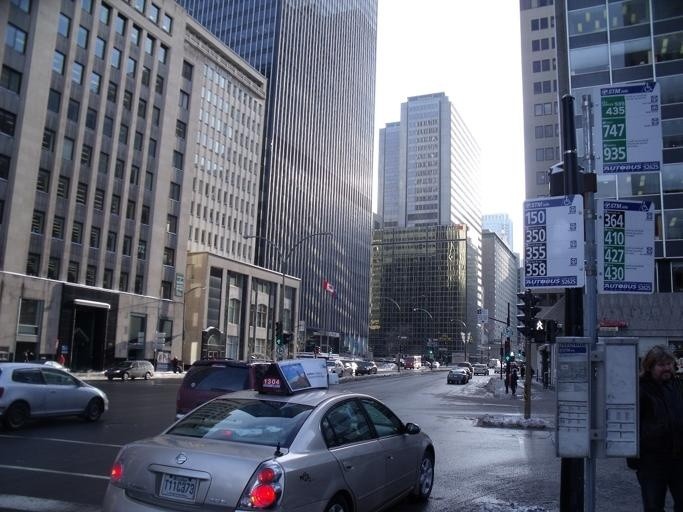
[494, 366, 505, 373]
[447, 361, 473, 384]
[0, 359, 110, 428]
[474, 364, 489, 375]
[378, 358, 404, 366]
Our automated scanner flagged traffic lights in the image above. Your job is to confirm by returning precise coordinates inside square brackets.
[275, 322, 282, 345]
[505, 341, 511, 363]
[516, 289, 556, 344]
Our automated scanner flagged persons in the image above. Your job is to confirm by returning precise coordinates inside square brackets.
[171, 356, 183, 373]
[505, 361, 534, 396]
[58, 353, 65, 366]
[624, 345, 683, 512]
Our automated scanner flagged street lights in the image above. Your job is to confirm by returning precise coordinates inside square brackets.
[375, 297, 401, 352]
[242, 232, 334, 361]
[413, 307, 432, 370]
[450, 319, 482, 361]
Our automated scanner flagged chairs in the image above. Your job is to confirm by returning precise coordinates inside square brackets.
[325, 412, 357, 442]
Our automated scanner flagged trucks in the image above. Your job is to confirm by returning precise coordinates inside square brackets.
[489, 359, 499, 369]
[404, 356, 421, 370]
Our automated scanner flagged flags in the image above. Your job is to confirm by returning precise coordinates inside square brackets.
[323, 280, 337, 299]
